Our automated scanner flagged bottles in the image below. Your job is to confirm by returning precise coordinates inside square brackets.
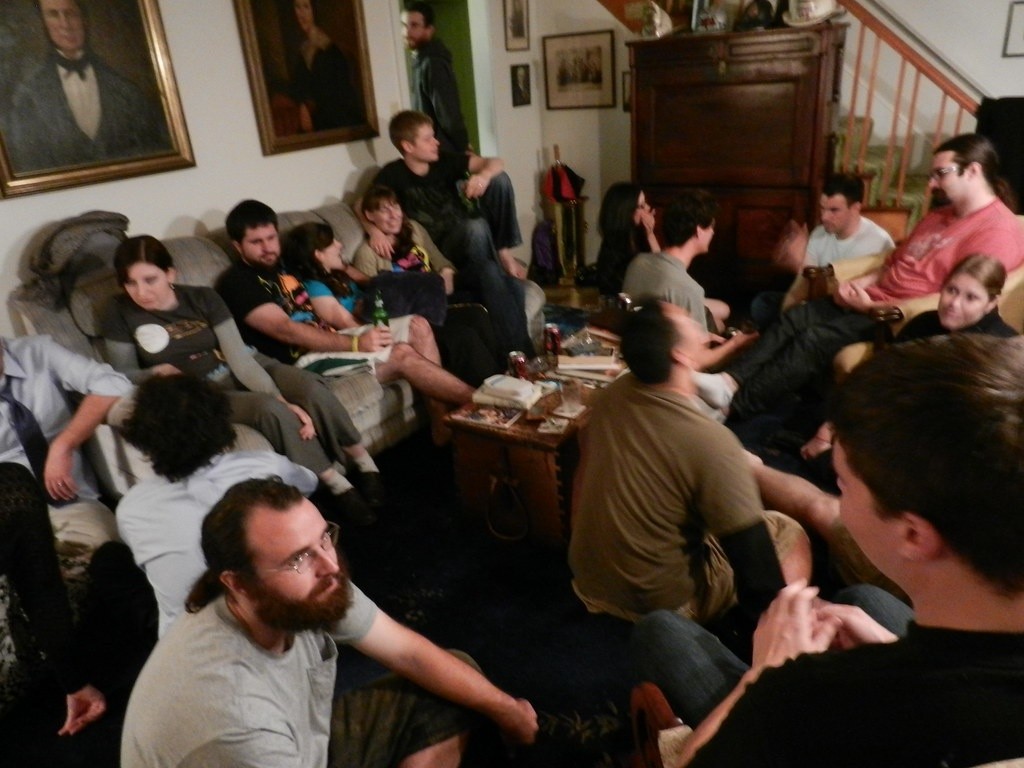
[374, 300, 389, 329]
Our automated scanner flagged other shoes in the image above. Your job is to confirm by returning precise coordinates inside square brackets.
[483, 467, 529, 541]
[356, 461, 391, 509]
[328, 488, 378, 532]
[426, 401, 454, 446]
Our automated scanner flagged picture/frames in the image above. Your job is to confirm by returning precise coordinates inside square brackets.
[541, 28, 619, 112]
[621, 70, 632, 113]
[1001, 0, 1024, 57]
[510, 63, 532, 107]
[0, 0, 198, 201]
[501, 0, 531, 52]
[232, 0, 382, 157]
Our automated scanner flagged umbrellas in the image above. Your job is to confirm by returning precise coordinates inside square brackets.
[544, 144, 585, 277]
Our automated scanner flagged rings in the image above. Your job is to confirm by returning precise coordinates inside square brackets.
[57, 482, 64, 486]
[478, 182, 482, 187]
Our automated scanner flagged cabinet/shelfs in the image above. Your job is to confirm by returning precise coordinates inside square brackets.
[624, 24, 846, 295]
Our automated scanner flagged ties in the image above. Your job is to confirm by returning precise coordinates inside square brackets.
[0, 377, 66, 509]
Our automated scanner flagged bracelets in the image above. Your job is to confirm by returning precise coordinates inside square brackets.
[351, 336, 359, 351]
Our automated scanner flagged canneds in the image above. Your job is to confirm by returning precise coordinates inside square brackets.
[507, 350, 527, 380]
[543, 323, 561, 355]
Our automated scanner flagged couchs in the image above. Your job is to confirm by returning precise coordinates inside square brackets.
[10, 236, 274, 492]
[204, 201, 422, 458]
[350, 189, 546, 357]
[785, 215, 1024, 391]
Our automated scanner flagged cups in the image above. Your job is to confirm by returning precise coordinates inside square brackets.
[559, 378, 581, 411]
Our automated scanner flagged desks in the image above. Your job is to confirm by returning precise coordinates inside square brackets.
[443, 326, 633, 535]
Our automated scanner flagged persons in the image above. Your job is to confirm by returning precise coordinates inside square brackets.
[0, 111, 1024, 768]
[401, 0, 473, 149]
